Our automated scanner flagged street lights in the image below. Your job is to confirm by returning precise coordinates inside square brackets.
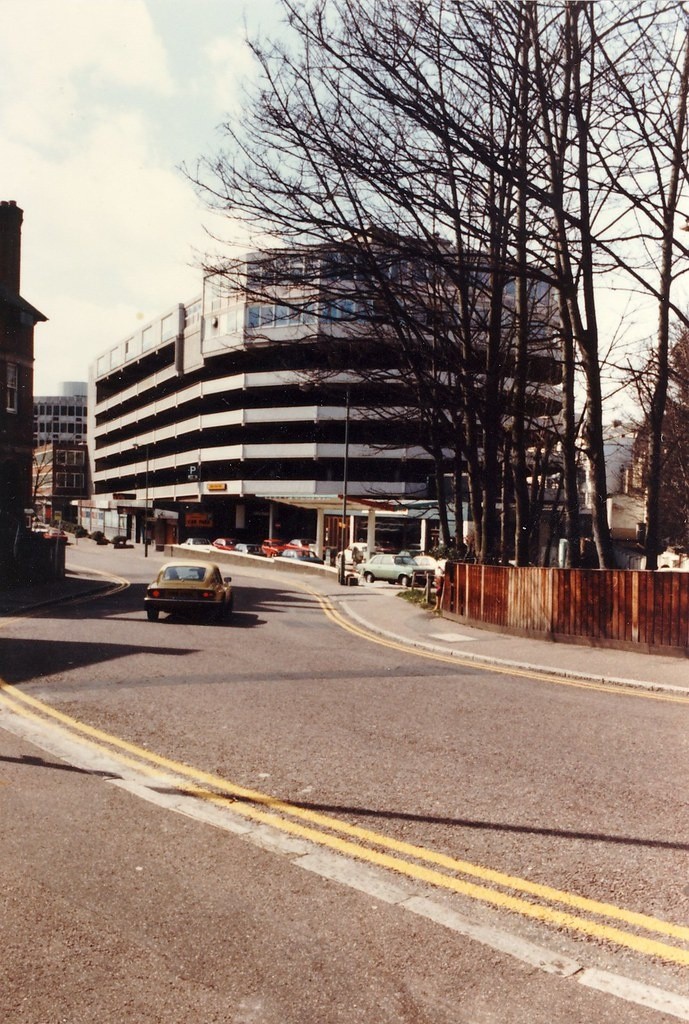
[297, 380, 350, 582]
[133, 442, 149, 556]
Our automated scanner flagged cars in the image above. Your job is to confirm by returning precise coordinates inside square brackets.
[44, 529, 68, 543]
[335, 541, 384, 572]
[181, 537, 214, 549]
[356, 553, 436, 589]
[144, 560, 235, 625]
[212, 537, 242, 552]
[262, 538, 287, 559]
[281, 536, 457, 587]
[234, 542, 266, 558]
